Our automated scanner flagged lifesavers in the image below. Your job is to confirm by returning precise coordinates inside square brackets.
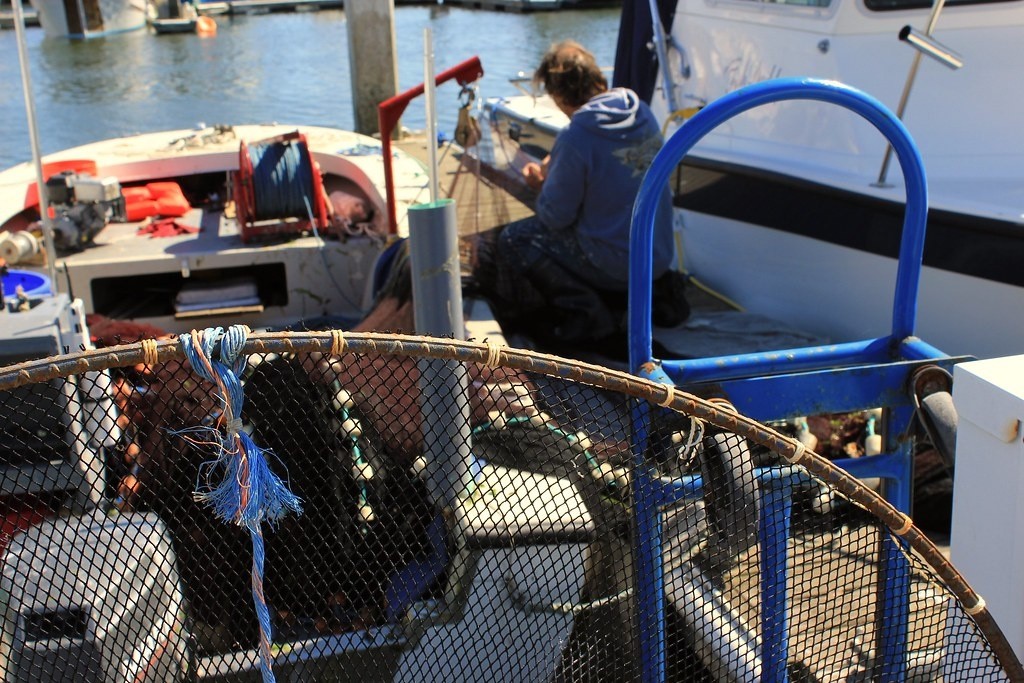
[696, 396, 759, 555]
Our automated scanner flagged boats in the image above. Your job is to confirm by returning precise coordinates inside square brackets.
[151, 2, 199, 34]
[488, 0, 1022, 358]
[0, 121, 594, 683]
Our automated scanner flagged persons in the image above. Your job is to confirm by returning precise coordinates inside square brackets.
[496, 40, 672, 349]
